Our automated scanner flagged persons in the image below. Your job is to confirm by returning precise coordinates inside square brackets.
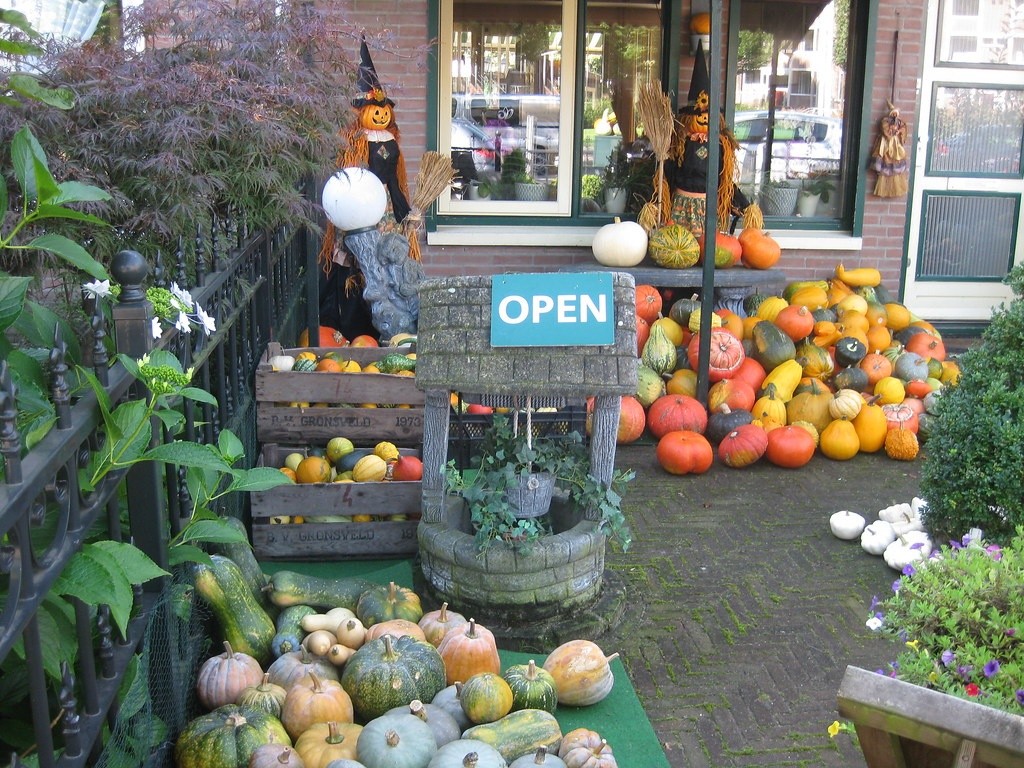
[637, 103, 763, 238]
[320, 93, 422, 274]
[376, 232, 425, 320]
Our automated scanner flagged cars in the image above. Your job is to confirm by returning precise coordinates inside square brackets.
[628, 109, 843, 185]
[452, 93, 560, 173]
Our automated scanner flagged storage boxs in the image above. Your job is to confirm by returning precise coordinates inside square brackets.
[251, 342, 427, 560]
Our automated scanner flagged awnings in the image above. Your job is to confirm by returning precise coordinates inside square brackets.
[455, 0, 664, 32]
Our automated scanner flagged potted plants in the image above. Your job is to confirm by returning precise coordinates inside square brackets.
[690, 12, 710, 51]
[761, 180, 798, 216]
[797, 169, 836, 217]
[439, 411, 638, 562]
[451, 148, 656, 213]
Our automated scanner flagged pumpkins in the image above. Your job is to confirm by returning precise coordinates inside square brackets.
[268, 328, 558, 413]
[830, 497, 931, 568]
[590, 219, 959, 467]
[176, 556, 619, 768]
[270, 439, 422, 522]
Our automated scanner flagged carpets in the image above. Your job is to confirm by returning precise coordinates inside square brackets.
[494, 648, 672, 768]
[257, 557, 415, 590]
